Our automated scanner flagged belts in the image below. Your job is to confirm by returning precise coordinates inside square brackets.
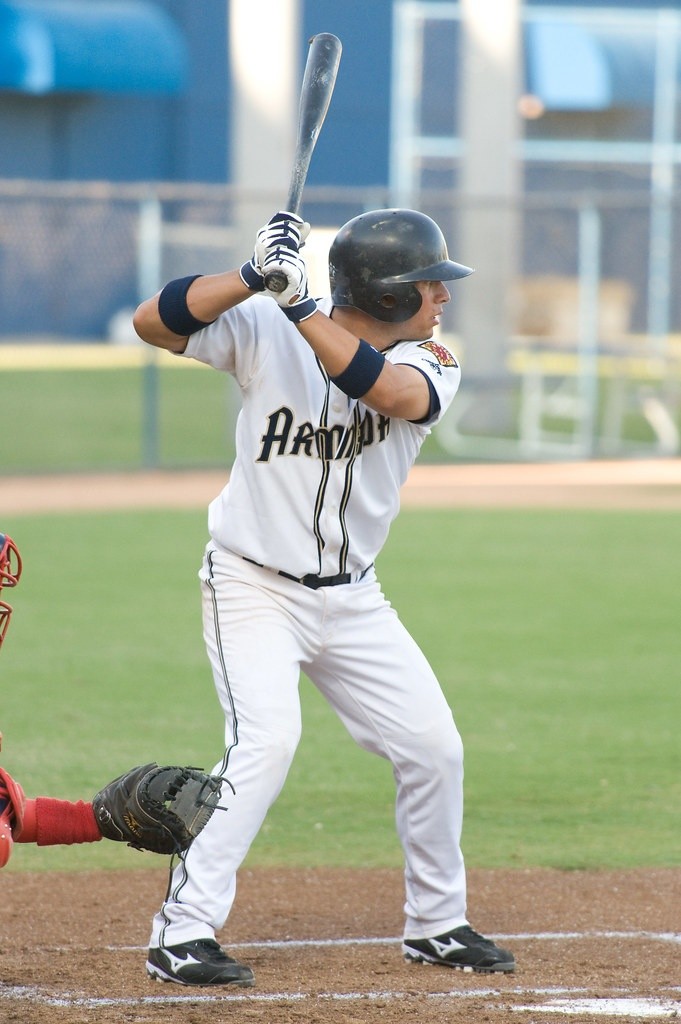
[243, 557, 373, 590]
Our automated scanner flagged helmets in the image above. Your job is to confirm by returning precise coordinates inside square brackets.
[328, 208, 475, 323]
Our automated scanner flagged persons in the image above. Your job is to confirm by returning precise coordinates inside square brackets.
[0, 533, 237, 870]
[132, 208, 514, 985]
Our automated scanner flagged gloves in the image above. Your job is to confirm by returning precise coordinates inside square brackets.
[239, 211, 312, 292]
[262, 248, 317, 322]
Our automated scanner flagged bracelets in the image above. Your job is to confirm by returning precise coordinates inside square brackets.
[328, 337, 386, 400]
[158, 274, 219, 337]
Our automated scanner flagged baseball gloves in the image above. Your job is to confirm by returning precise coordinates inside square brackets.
[89, 758, 227, 858]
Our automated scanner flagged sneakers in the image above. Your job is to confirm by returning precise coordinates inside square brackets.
[146, 938, 255, 988]
[401, 925, 515, 974]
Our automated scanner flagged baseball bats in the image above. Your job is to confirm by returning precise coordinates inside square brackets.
[261, 28, 345, 296]
